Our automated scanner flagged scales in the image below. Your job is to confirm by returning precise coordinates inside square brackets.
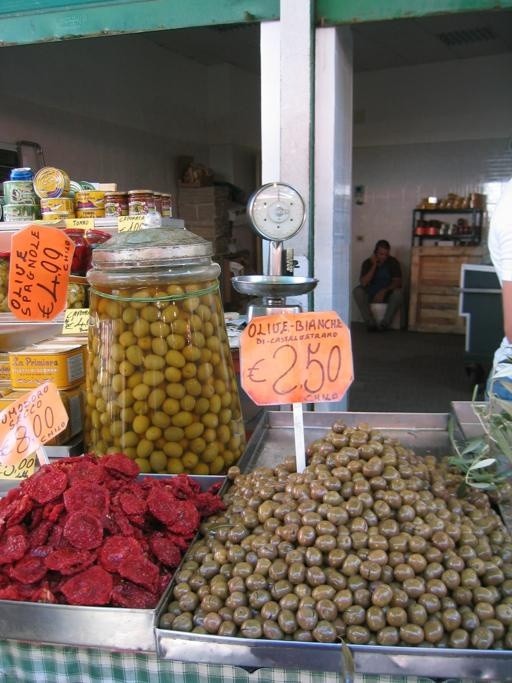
[231, 182, 320, 324]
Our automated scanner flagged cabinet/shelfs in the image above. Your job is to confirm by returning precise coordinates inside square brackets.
[224, 250, 250, 304]
[410, 209, 484, 249]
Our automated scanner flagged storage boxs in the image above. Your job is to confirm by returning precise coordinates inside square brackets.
[369, 302, 404, 329]
[178, 186, 233, 253]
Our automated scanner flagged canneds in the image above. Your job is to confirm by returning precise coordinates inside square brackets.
[0, 166, 173, 221]
[62, 229, 111, 309]
[0, 333, 88, 446]
[0, 251, 13, 313]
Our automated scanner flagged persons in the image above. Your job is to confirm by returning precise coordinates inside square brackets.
[353, 239, 405, 332]
[484, 177, 511, 401]
